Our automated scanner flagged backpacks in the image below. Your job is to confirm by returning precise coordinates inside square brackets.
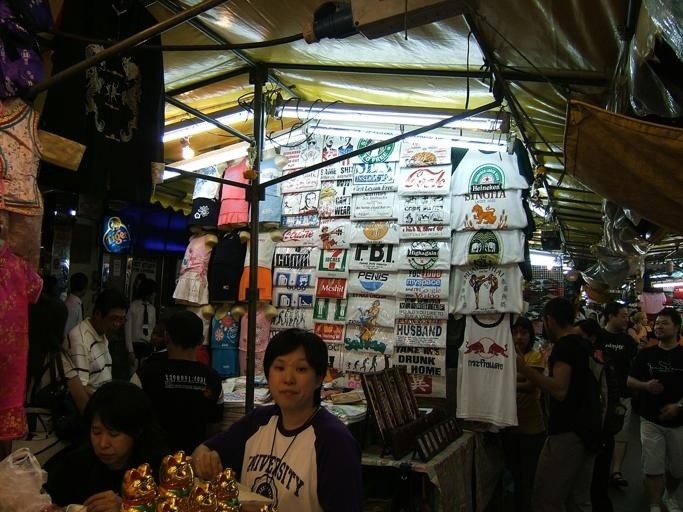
[591, 362, 629, 438]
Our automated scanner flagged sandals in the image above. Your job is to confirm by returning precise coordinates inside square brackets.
[610, 471, 629, 487]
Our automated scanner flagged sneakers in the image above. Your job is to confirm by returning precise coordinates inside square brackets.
[661, 494, 680, 512]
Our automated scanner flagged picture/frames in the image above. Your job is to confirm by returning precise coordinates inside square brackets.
[359, 364, 418, 455]
[415, 417, 464, 460]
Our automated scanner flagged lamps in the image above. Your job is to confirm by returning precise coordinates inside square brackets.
[178, 138, 195, 159]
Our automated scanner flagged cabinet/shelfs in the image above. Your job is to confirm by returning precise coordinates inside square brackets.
[353, 431, 481, 511]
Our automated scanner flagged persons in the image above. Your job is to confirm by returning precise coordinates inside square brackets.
[499, 298, 682, 510]
[189, 330, 366, 512]
[37, 261, 223, 510]
